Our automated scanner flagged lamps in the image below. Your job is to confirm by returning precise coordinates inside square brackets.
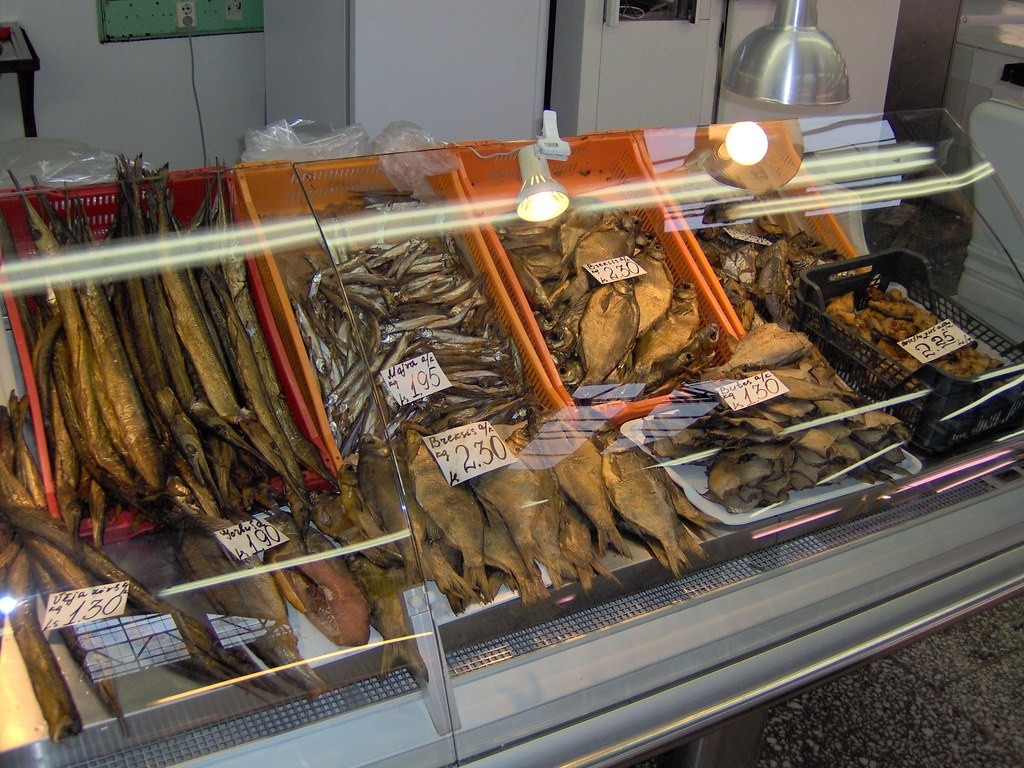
[680, 117, 803, 191]
[725, 0, 851, 106]
[448, 140, 569, 223]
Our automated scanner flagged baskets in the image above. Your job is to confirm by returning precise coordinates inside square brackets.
[440, 129, 743, 423]
[789, 247, 1024, 460]
[233, 141, 569, 481]
[634, 119, 867, 336]
[0, 164, 341, 548]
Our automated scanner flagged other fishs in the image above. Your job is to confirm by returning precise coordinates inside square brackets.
[0, 149, 1009, 750]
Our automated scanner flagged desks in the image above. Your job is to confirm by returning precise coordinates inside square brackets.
[0, 21, 40, 138]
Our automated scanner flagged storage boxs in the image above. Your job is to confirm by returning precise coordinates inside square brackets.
[0, 164, 340, 546]
[760, 232, 1023, 457]
[634, 119, 869, 338]
[448, 129, 742, 425]
[234, 142, 573, 475]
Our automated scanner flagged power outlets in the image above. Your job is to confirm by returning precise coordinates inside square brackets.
[177, 1, 199, 28]
